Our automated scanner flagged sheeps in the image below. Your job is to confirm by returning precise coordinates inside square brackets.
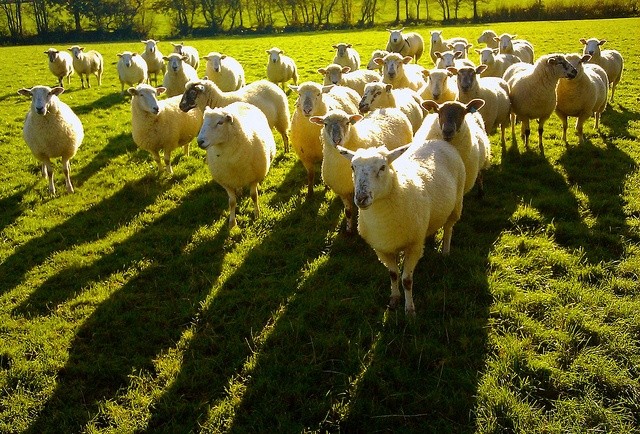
[307, 105, 416, 237]
[366, 50, 391, 75]
[336, 137, 468, 318]
[474, 47, 523, 78]
[202, 51, 245, 92]
[358, 80, 430, 136]
[194, 99, 278, 232]
[413, 98, 492, 199]
[178, 75, 292, 153]
[116, 51, 149, 95]
[316, 63, 382, 99]
[161, 52, 200, 98]
[140, 38, 167, 87]
[16, 83, 85, 195]
[332, 42, 362, 73]
[265, 47, 298, 92]
[502, 53, 578, 149]
[44, 47, 74, 87]
[429, 30, 468, 65]
[170, 40, 200, 72]
[446, 63, 511, 143]
[67, 45, 104, 89]
[492, 34, 535, 65]
[579, 37, 625, 103]
[385, 28, 424, 64]
[477, 30, 534, 50]
[433, 50, 476, 70]
[419, 68, 459, 104]
[446, 41, 473, 59]
[373, 51, 427, 92]
[286, 80, 363, 198]
[125, 82, 204, 178]
[554, 53, 610, 148]
